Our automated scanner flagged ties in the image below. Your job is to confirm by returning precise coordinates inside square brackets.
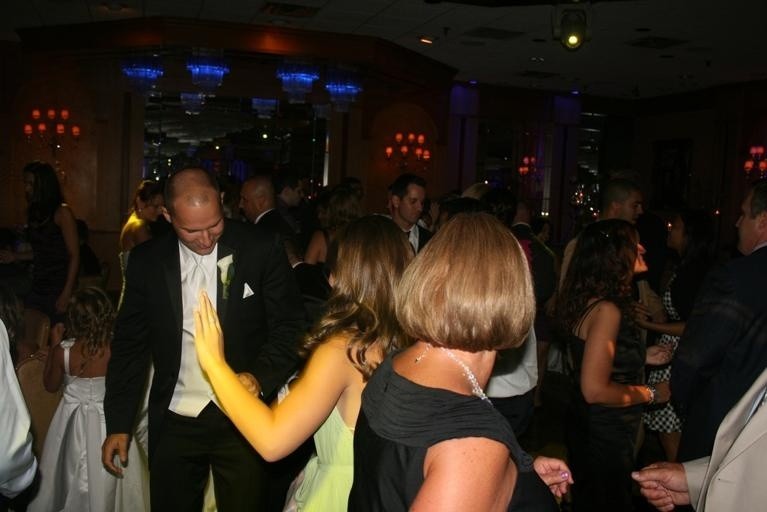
[190, 254, 206, 291]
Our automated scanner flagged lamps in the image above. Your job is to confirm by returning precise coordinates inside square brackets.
[743, 146, 767, 179]
[275, 64, 320, 103]
[23, 108, 83, 166]
[386, 130, 431, 174]
[119, 60, 165, 96]
[323, 71, 363, 112]
[179, 91, 205, 116]
[251, 97, 278, 119]
[559, 9, 584, 52]
[185, 59, 230, 97]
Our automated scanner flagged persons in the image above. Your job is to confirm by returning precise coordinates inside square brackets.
[631, 368, 767, 510]
[1, 164, 717, 511]
[669, 179, 766, 511]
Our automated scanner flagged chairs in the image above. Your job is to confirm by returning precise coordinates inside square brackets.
[14, 351, 64, 461]
[17, 308, 50, 367]
